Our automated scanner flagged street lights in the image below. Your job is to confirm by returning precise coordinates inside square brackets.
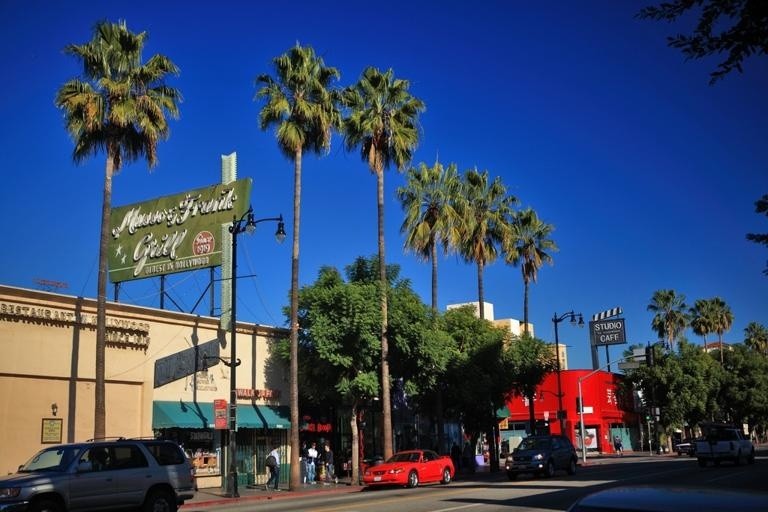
[222, 205, 286, 497]
[539, 310, 584, 439]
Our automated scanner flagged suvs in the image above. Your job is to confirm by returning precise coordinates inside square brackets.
[0, 439, 197, 512]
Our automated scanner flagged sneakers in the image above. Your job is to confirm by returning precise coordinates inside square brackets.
[307, 477, 339, 485]
[265, 484, 270, 489]
[273, 489, 280, 492]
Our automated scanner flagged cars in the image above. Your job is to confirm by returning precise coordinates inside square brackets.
[504, 435, 578, 481]
[674, 438, 697, 457]
[363, 450, 455, 490]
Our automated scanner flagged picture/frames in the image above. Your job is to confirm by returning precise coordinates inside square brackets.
[41, 419, 63, 443]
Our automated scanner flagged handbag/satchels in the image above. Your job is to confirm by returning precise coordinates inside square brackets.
[265, 456, 276, 466]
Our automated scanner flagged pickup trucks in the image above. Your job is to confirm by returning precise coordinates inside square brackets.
[694, 429, 754, 468]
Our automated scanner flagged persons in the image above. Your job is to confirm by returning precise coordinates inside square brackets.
[80, 449, 104, 472]
[264, 445, 281, 491]
[615, 436, 623, 455]
[451, 441, 461, 471]
[502, 437, 508, 460]
[463, 443, 472, 463]
[301, 440, 338, 485]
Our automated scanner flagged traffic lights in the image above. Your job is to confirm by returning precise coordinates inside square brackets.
[645, 346, 655, 367]
[646, 415, 654, 420]
